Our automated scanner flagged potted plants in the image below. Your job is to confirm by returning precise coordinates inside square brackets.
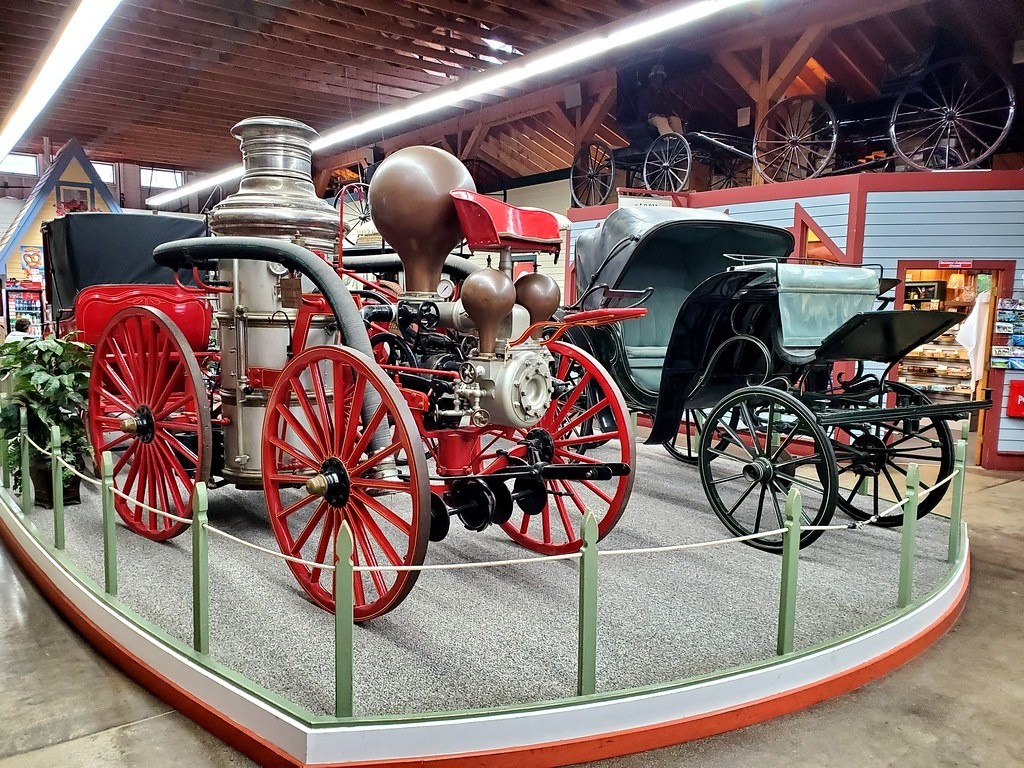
[1, 329, 96, 509]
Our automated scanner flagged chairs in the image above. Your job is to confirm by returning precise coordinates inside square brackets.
[615, 266, 709, 367]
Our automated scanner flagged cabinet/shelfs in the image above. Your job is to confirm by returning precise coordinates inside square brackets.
[897, 280, 975, 402]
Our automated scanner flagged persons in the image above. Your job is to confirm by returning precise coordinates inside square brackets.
[4, 318, 35, 343]
